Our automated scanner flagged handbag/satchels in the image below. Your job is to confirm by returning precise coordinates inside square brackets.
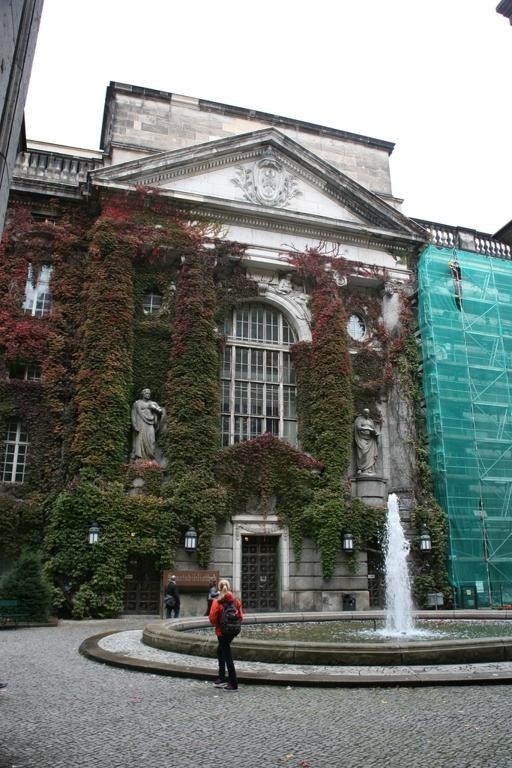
[164, 594, 176, 607]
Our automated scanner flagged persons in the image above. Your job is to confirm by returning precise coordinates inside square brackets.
[163, 576, 180, 620]
[130, 389, 162, 460]
[204, 577, 220, 616]
[209, 579, 244, 693]
[354, 408, 381, 475]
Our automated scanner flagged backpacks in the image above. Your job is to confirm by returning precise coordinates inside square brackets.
[220, 599, 241, 637]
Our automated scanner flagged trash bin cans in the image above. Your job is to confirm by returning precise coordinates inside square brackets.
[343, 594, 356, 611]
[461, 585, 478, 610]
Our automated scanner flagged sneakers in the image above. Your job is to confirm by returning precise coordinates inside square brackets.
[215, 678, 237, 691]
